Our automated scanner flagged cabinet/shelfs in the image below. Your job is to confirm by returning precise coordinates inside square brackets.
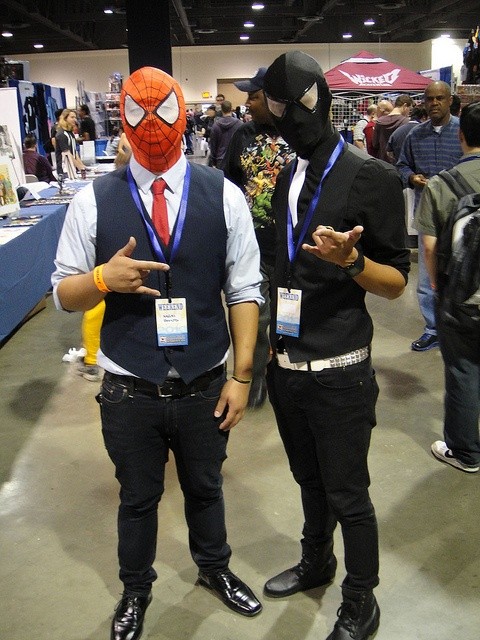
[85, 90, 106, 140]
[105, 93, 120, 137]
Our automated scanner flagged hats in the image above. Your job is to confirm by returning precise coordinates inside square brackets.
[233, 67, 267, 92]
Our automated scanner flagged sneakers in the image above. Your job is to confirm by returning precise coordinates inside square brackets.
[431, 440, 480, 473]
[75, 358, 100, 383]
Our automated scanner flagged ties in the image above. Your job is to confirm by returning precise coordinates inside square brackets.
[151, 178, 170, 247]
[288, 152, 309, 228]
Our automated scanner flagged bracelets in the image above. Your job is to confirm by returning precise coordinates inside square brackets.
[93, 264, 113, 295]
[229, 376, 254, 384]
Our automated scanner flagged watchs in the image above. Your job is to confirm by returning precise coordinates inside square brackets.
[339, 252, 365, 278]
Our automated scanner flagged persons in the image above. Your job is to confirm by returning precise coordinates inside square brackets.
[55, 109, 83, 176]
[208, 102, 243, 163]
[375, 95, 415, 161]
[354, 105, 379, 150]
[184, 108, 195, 155]
[260, 50, 411, 632]
[24, 135, 49, 181]
[418, 101, 478, 474]
[51, 66, 264, 640]
[78, 104, 95, 142]
[400, 82, 464, 210]
[204, 106, 219, 125]
[214, 93, 227, 107]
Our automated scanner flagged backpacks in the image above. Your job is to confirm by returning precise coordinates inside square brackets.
[437, 165, 480, 335]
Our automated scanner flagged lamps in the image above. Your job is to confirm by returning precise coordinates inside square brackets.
[368, 26, 391, 35]
[363, 17, 376, 26]
[239, 33, 251, 40]
[196, 21, 219, 35]
[374, 0, 406, 10]
[103, 8, 113, 14]
[278, 36, 298, 43]
[1, 30, 14, 37]
[251, 1, 265, 10]
[33, 41, 44, 49]
[5, 18, 32, 30]
[243, 19, 254, 28]
[296, 12, 323, 23]
[342, 31, 353, 39]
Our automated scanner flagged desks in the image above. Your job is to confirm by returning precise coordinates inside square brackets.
[0, 204, 67, 339]
[18, 182, 91, 205]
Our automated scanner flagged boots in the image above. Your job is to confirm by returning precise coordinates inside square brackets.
[324, 581, 380, 640]
[265, 538, 336, 598]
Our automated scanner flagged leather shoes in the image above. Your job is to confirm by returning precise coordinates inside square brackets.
[111, 587, 152, 640]
[411, 332, 439, 350]
[199, 566, 262, 617]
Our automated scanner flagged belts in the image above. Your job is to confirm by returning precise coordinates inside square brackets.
[104, 364, 225, 398]
[275, 346, 369, 371]
